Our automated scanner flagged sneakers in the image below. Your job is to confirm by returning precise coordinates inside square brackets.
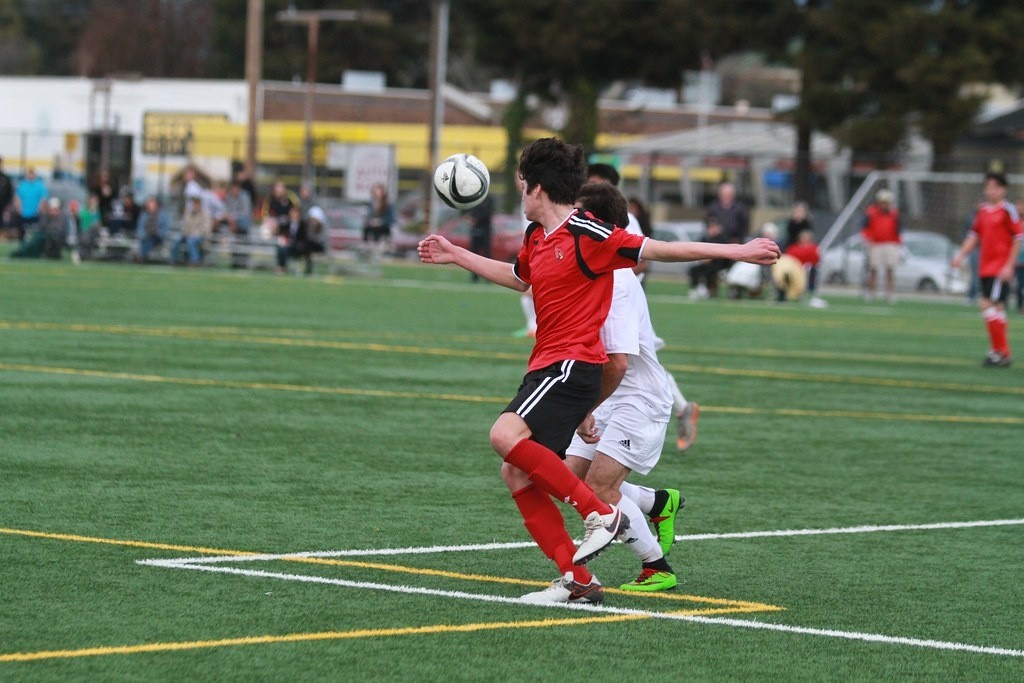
[572, 503, 630, 566]
[521, 570, 603, 605]
[620, 561, 677, 592]
[649, 488, 686, 557]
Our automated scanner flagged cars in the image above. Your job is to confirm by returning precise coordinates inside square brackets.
[820, 232, 974, 295]
[646, 224, 717, 280]
[435, 213, 526, 263]
[324, 208, 420, 261]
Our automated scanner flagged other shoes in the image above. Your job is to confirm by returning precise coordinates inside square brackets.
[983, 352, 1013, 368]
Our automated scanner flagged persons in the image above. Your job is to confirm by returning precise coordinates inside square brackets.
[862, 189, 907, 304]
[417, 139, 782, 603]
[573, 183, 684, 593]
[0, 158, 328, 277]
[685, 180, 746, 301]
[948, 171, 1023, 368]
[724, 202, 819, 302]
[361, 184, 392, 245]
[511, 164, 700, 450]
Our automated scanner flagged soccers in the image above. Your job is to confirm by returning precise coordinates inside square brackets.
[433, 153, 490, 210]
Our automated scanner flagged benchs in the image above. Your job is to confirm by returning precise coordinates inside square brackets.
[64, 227, 384, 280]
